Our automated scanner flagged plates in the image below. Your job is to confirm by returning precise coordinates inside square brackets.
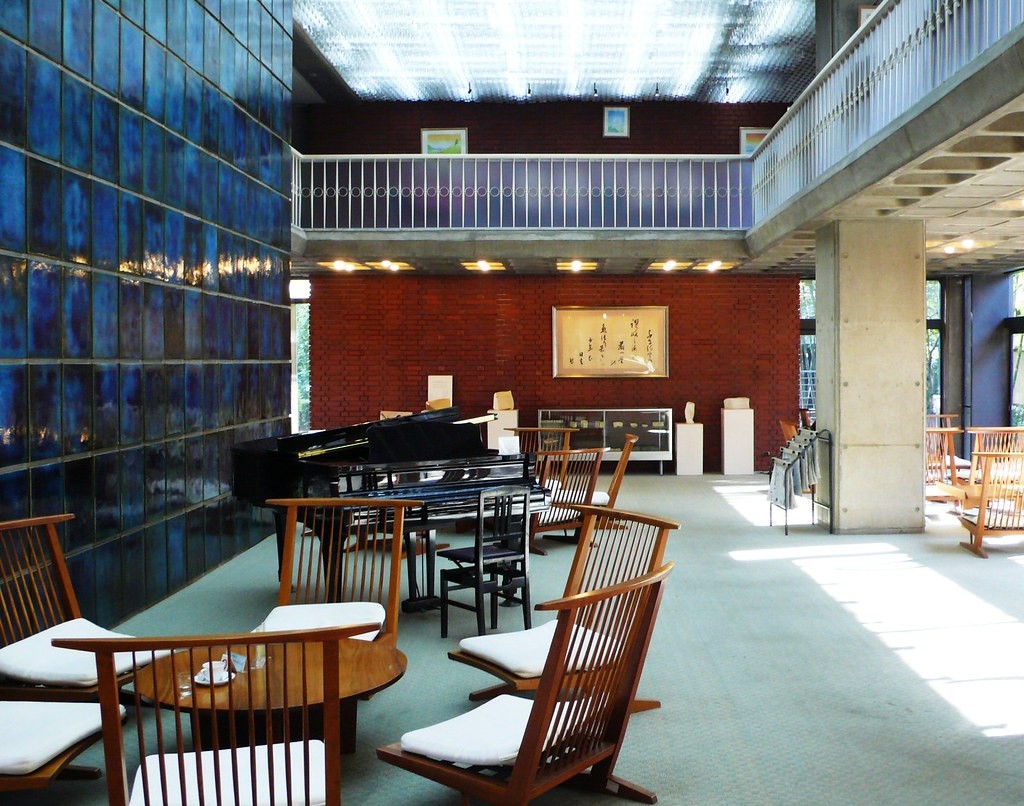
[194, 672, 235, 686]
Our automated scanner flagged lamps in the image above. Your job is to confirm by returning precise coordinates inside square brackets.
[527, 84, 532, 95]
[725, 81, 731, 95]
[593, 83, 599, 97]
[467, 82, 473, 94]
[654, 83, 661, 97]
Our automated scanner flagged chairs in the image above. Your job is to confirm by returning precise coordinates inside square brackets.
[376, 559, 679, 806]
[503, 427, 583, 489]
[253, 497, 428, 652]
[0, 512, 177, 707]
[446, 500, 683, 718]
[927, 412, 1024, 560]
[778, 421, 815, 493]
[493, 439, 604, 555]
[439, 480, 535, 640]
[554, 433, 639, 532]
[0, 684, 127, 790]
[48, 611, 385, 806]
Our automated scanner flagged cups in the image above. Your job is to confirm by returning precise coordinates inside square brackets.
[178, 671, 193, 696]
[200, 661, 224, 682]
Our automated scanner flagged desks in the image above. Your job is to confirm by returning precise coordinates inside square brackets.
[133, 637, 412, 758]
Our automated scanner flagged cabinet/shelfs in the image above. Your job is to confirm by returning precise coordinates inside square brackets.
[536, 408, 672, 477]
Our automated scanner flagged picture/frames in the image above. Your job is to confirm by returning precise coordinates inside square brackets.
[740, 127, 772, 155]
[551, 305, 671, 380]
[604, 106, 630, 138]
[420, 127, 469, 155]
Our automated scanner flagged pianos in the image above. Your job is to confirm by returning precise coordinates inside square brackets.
[230, 405, 552, 613]
